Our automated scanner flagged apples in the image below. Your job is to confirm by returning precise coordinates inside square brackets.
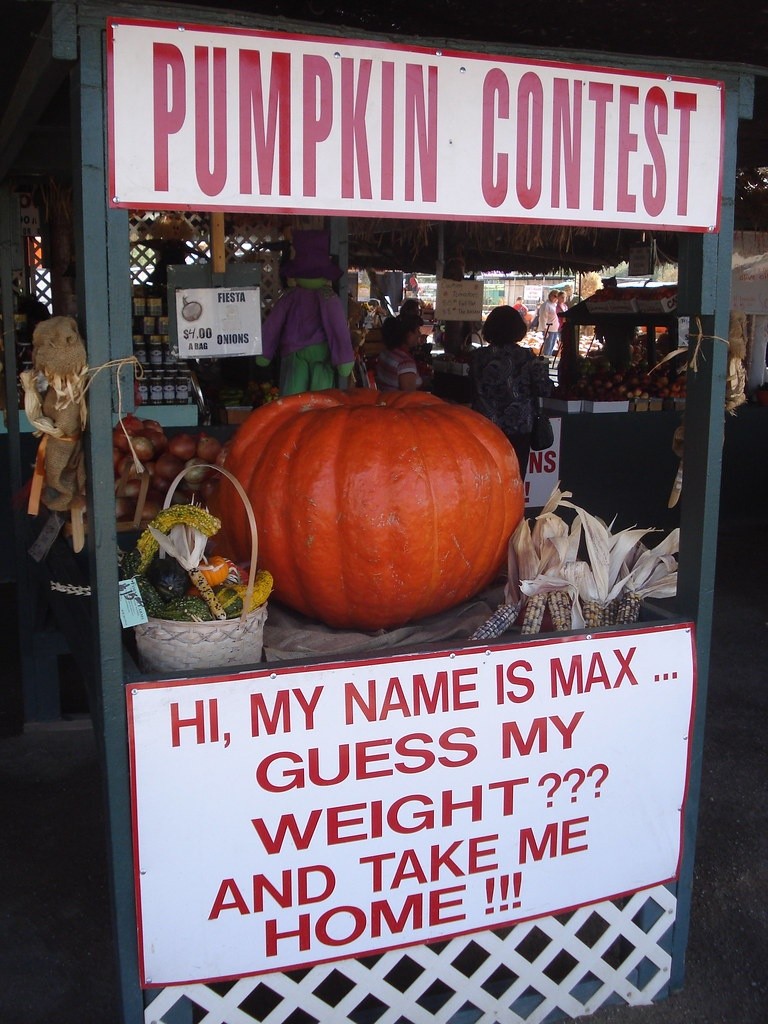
[551, 373, 687, 401]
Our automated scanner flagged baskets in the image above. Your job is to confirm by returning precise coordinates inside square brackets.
[457, 332, 483, 363]
[133, 465, 268, 675]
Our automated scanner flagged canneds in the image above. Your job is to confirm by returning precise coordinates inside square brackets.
[132, 297, 193, 405]
[14, 341, 34, 409]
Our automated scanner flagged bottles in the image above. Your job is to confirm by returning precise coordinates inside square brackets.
[131, 298, 193, 406]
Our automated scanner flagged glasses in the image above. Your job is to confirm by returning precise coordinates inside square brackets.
[553, 294, 557, 298]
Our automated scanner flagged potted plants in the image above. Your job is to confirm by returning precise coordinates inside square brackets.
[755, 382, 768, 407]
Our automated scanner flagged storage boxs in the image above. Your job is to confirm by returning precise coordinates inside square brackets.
[663, 397, 686, 411]
[543, 398, 582, 414]
[637, 293, 677, 313]
[648, 398, 663, 411]
[432, 359, 448, 374]
[582, 400, 629, 414]
[448, 361, 470, 375]
[218, 406, 254, 425]
[209, 402, 224, 423]
[629, 399, 649, 412]
[584, 297, 640, 314]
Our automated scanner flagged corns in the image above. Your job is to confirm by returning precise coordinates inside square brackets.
[468, 590, 641, 641]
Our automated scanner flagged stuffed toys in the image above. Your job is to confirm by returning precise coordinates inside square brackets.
[254, 230, 356, 398]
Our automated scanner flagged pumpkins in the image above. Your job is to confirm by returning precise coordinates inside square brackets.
[217, 387, 525, 630]
[121, 503, 273, 621]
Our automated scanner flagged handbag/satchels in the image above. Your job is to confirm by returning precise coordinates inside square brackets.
[531, 414, 554, 451]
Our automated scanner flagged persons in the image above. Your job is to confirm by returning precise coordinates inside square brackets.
[514, 283, 584, 356]
[370, 298, 433, 393]
[469, 305, 555, 481]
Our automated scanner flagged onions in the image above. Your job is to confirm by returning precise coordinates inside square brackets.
[108, 416, 231, 520]
